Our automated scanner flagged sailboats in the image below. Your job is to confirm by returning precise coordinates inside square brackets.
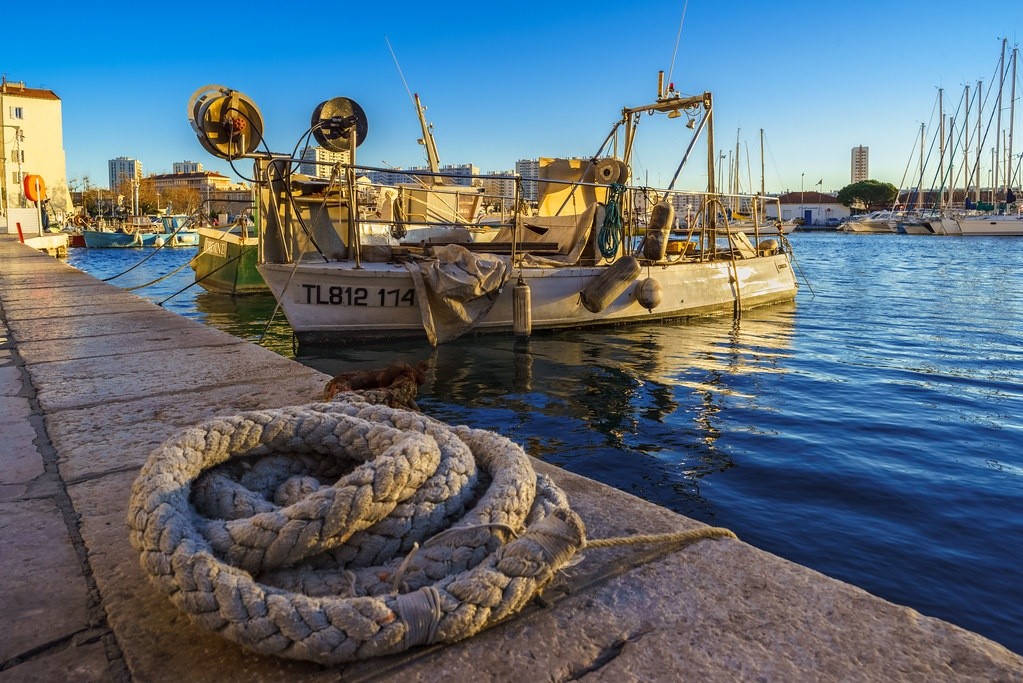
[835, 33, 1023, 236]
[626, 126, 799, 234]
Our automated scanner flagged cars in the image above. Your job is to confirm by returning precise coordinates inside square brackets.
[793, 217, 806, 226]
[840, 216, 855, 225]
[826, 218, 840, 227]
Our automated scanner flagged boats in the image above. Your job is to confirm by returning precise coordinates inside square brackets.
[188, 0, 815, 350]
[189, 199, 273, 297]
[69, 179, 203, 248]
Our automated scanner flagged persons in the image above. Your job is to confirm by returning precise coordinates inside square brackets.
[34, 198, 54, 235]
[1006, 187, 1015, 214]
[675, 217, 678, 229]
